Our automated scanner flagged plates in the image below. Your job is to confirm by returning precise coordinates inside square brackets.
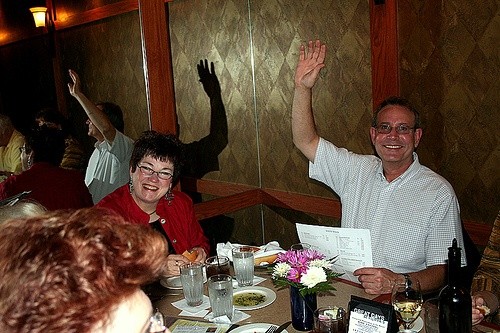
[399, 316, 423, 333]
[232, 286, 277, 310]
[160, 275, 206, 290]
[227, 323, 289, 333]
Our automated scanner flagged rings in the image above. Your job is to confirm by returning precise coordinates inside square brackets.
[176, 261, 179, 266]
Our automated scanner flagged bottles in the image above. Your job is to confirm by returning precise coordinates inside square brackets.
[335, 308, 346, 333]
[437, 238, 473, 333]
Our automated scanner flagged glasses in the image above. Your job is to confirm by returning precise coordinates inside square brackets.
[137, 163, 174, 181]
[19, 146, 26, 153]
[374, 124, 418, 134]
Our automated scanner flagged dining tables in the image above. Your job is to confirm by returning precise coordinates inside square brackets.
[155, 240, 500, 333]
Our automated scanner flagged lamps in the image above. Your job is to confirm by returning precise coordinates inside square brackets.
[29, 7, 47, 32]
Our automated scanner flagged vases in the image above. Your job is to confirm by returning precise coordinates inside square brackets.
[291, 288, 318, 330]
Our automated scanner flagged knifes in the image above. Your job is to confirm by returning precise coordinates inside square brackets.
[273, 321, 292, 333]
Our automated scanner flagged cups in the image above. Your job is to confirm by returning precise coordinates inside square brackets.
[179, 261, 204, 306]
[424, 299, 439, 333]
[207, 273, 234, 321]
[391, 283, 424, 333]
[204, 256, 231, 281]
[232, 246, 255, 287]
[313, 308, 331, 333]
[290, 242, 312, 252]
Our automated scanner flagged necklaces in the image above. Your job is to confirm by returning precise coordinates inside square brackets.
[149, 210, 157, 215]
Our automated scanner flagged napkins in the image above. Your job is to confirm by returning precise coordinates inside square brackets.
[216, 241, 284, 261]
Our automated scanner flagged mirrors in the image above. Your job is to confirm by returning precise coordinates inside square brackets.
[0, 0, 176, 210]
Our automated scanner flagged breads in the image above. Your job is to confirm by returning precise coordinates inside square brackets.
[255, 254, 278, 264]
[182, 249, 199, 261]
[475, 305, 490, 316]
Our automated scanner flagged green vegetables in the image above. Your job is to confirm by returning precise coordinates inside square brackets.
[235, 293, 266, 306]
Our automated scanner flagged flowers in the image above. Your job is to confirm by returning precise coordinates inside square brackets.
[271, 246, 333, 287]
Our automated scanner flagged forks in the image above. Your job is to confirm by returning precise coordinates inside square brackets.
[264, 326, 277, 333]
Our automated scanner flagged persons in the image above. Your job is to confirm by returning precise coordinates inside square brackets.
[86, 131, 209, 303]
[469, 211, 500, 327]
[0, 200, 48, 223]
[66, 68, 135, 205]
[0, 106, 94, 211]
[0, 209, 175, 333]
[290, 40, 467, 304]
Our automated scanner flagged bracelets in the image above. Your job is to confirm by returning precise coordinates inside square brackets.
[404, 272, 411, 294]
